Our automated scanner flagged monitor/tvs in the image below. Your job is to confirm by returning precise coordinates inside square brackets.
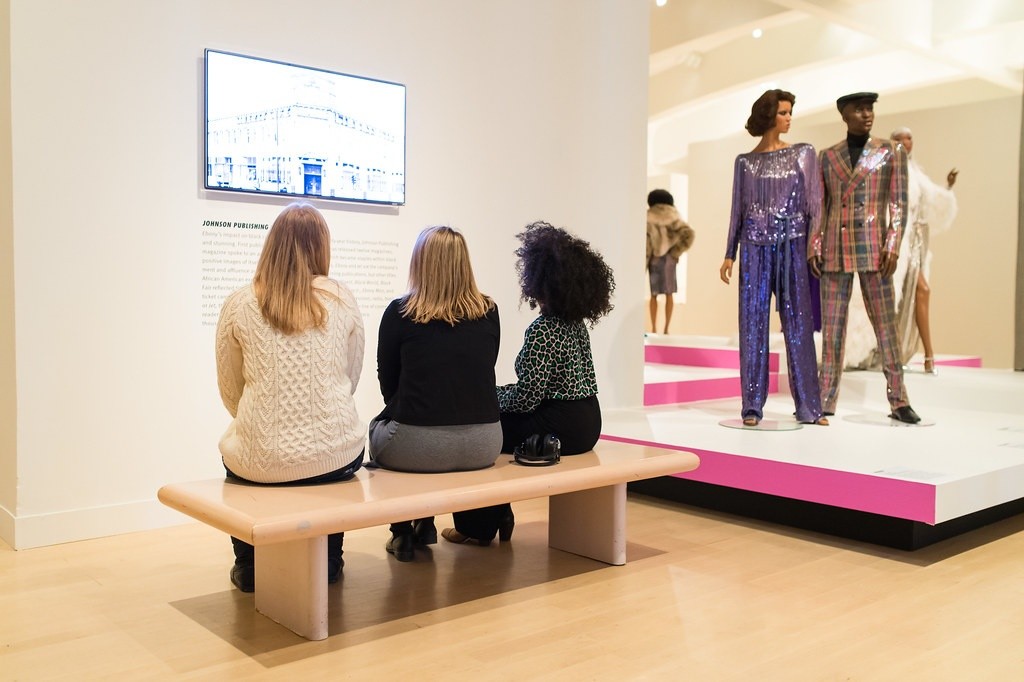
[202, 47, 408, 206]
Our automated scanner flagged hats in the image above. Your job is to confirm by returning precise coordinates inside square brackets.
[837, 92, 879, 112]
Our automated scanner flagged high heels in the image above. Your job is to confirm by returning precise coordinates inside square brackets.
[441, 514, 515, 545]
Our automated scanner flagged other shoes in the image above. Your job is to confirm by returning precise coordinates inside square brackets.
[230, 561, 255, 592]
[892, 406, 920, 423]
[415, 521, 439, 545]
[328, 555, 345, 582]
[386, 531, 416, 562]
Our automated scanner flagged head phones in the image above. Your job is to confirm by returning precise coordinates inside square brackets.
[513, 433, 562, 466]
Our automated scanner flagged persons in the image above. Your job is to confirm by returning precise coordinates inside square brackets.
[845, 126, 960, 373]
[819, 91, 921, 424]
[720, 89, 828, 426]
[216, 204, 366, 593]
[441, 221, 616, 547]
[645, 189, 695, 334]
[369, 225, 504, 562]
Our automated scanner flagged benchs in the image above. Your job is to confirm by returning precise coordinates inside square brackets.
[158, 439, 700, 641]
